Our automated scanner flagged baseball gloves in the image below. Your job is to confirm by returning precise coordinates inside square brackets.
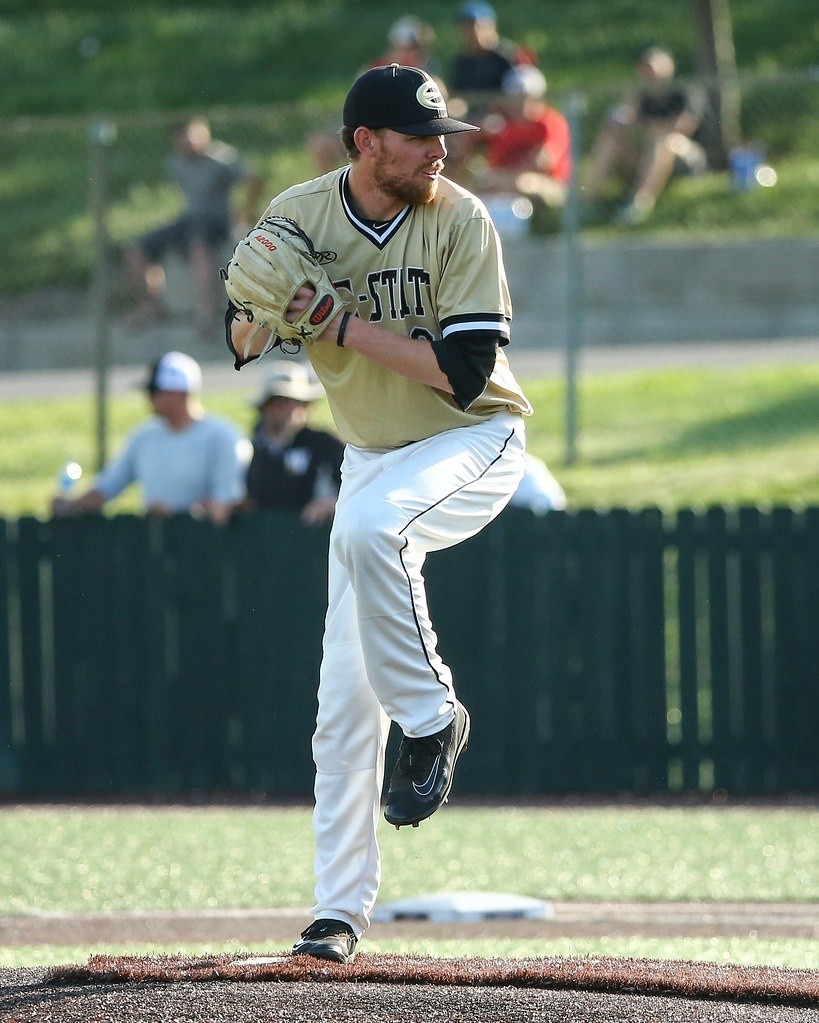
[224, 215, 344, 346]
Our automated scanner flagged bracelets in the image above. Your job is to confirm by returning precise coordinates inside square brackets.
[336, 311, 352, 347]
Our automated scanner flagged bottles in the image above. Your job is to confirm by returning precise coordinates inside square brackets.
[57, 462, 77, 518]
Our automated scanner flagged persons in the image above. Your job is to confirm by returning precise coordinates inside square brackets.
[52, 352, 253, 523]
[462, 64, 572, 208]
[124, 118, 261, 333]
[586, 45, 724, 223]
[245, 361, 345, 526]
[373, 18, 436, 67]
[447, 0, 537, 94]
[217, 63, 534, 963]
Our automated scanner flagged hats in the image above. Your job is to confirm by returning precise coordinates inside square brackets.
[249, 361, 327, 406]
[131, 351, 201, 394]
[385, 18, 439, 48]
[505, 65, 548, 98]
[459, 3, 496, 23]
[342, 63, 482, 136]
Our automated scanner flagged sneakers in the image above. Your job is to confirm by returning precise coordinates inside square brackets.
[384, 699, 470, 830]
[291, 920, 358, 964]
